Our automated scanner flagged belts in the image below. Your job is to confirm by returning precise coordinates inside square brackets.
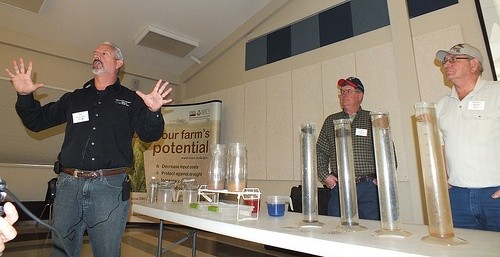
[62, 167, 125, 180]
[356, 175, 372, 184]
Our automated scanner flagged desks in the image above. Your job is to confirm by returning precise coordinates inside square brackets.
[128, 191, 500, 256]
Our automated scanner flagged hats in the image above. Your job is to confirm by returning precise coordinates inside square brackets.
[338, 77, 364, 94]
[436, 44, 484, 66]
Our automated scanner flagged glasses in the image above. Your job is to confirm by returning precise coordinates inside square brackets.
[442, 56, 468, 64]
[341, 89, 361, 95]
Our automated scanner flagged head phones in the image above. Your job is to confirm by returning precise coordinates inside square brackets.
[83, 77, 120, 98]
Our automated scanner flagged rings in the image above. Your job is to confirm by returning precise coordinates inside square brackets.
[161, 94, 164, 99]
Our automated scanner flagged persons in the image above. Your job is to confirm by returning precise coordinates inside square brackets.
[435, 44, 500, 233]
[5, 40, 172, 257]
[0, 202, 19, 257]
[316, 77, 398, 222]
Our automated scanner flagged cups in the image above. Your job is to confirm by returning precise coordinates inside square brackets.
[244, 195, 260, 214]
[159, 188, 173, 205]
[183, 190, 198, 206]
[207, 142, 248, 192]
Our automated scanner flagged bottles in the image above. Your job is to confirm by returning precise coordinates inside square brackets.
[146, 177, 157, 205]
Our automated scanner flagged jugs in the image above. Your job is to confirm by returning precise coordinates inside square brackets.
[264, 195, 294, 216]
